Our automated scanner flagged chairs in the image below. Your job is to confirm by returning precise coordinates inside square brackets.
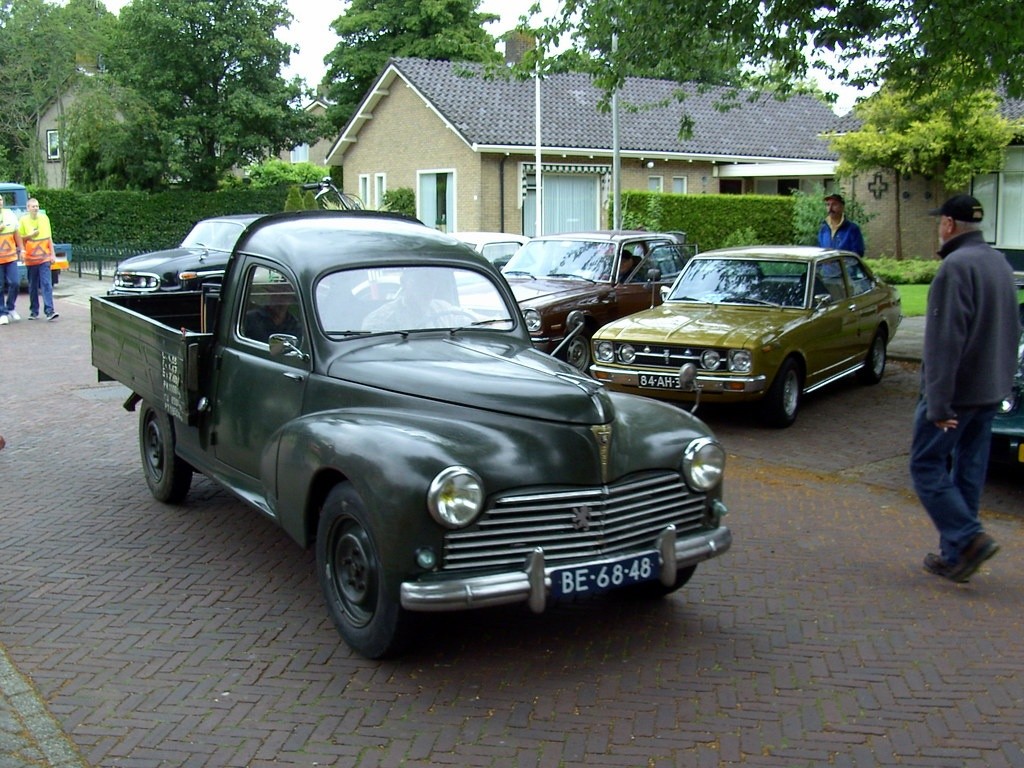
[632, 255, 644, 281]
[793, 273, 835, 306]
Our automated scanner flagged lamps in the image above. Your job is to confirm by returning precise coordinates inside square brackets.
[642, 160, 655, 169]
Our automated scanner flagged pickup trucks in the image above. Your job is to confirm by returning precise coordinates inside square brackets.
[88, 209, 731, 660]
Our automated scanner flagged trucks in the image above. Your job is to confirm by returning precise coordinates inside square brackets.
[0, 182, 73, 293]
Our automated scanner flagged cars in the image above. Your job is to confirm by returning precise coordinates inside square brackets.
[351, 231, 535, 319]
[589, 245, 905, 428]
[459, 231, 694, 373]
[989, 323, 1024, 476]
[104, 214, 289, 295]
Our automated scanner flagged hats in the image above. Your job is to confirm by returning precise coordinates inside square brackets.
[825, 194, 845, 205]
[928, 195, 984, 222]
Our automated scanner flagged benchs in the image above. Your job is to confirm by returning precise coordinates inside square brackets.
[760, 278, 847, 301]
[245, 300, 392, 345]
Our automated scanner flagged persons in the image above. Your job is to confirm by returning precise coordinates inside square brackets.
[818, 193, 865, 300]
[0, 194, 26, 325]
[361, 268, 504, 331]
[244, 274, 303, 344]
[19, 196, 60, 321]
[634, 243, 644, 260]
[600, 251, 642, 284]
[909, 196, 1023, 582]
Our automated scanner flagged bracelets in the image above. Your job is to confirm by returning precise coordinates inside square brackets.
[18, 249, 26, 255]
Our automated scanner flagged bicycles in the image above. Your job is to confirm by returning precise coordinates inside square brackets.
[303, 177, 364, 210]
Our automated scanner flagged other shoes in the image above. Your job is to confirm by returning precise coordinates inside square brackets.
[28, 312, 38, 319]
[0, 315, 9, 325]
[8, 310, 21, 321]
[47, 312, 58, 320]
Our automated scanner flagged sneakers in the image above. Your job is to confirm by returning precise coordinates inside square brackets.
[950, 532, 999, 581]
[924, 553, 947, 575]
